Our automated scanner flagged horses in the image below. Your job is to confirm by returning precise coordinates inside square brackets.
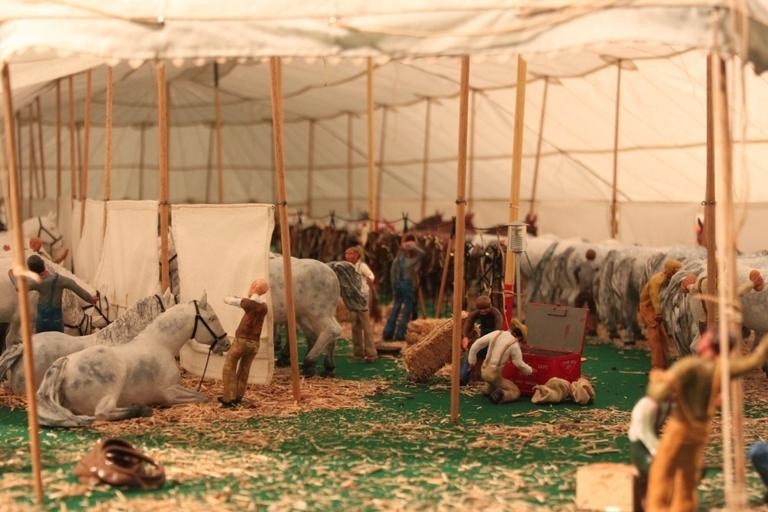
[0, 209, 231, 429]
